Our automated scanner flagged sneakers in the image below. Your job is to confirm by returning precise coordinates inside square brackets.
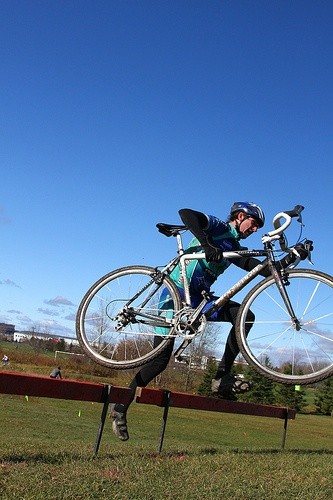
[211, 376, 250, 394]
[111, 405, 130, 441]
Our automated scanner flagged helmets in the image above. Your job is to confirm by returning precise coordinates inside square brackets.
[230, 201, 265, 228]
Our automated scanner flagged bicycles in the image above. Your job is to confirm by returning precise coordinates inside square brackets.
[74, 203, 333, 387]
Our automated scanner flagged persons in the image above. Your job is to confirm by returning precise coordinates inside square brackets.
[2, 354, 9, 366]
[110, 202, 313, 441]
[50, 366, 63, 379]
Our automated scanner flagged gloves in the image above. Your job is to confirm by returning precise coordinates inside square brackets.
[292, 240, 309, 263]
[202, 242, 223, 263]
